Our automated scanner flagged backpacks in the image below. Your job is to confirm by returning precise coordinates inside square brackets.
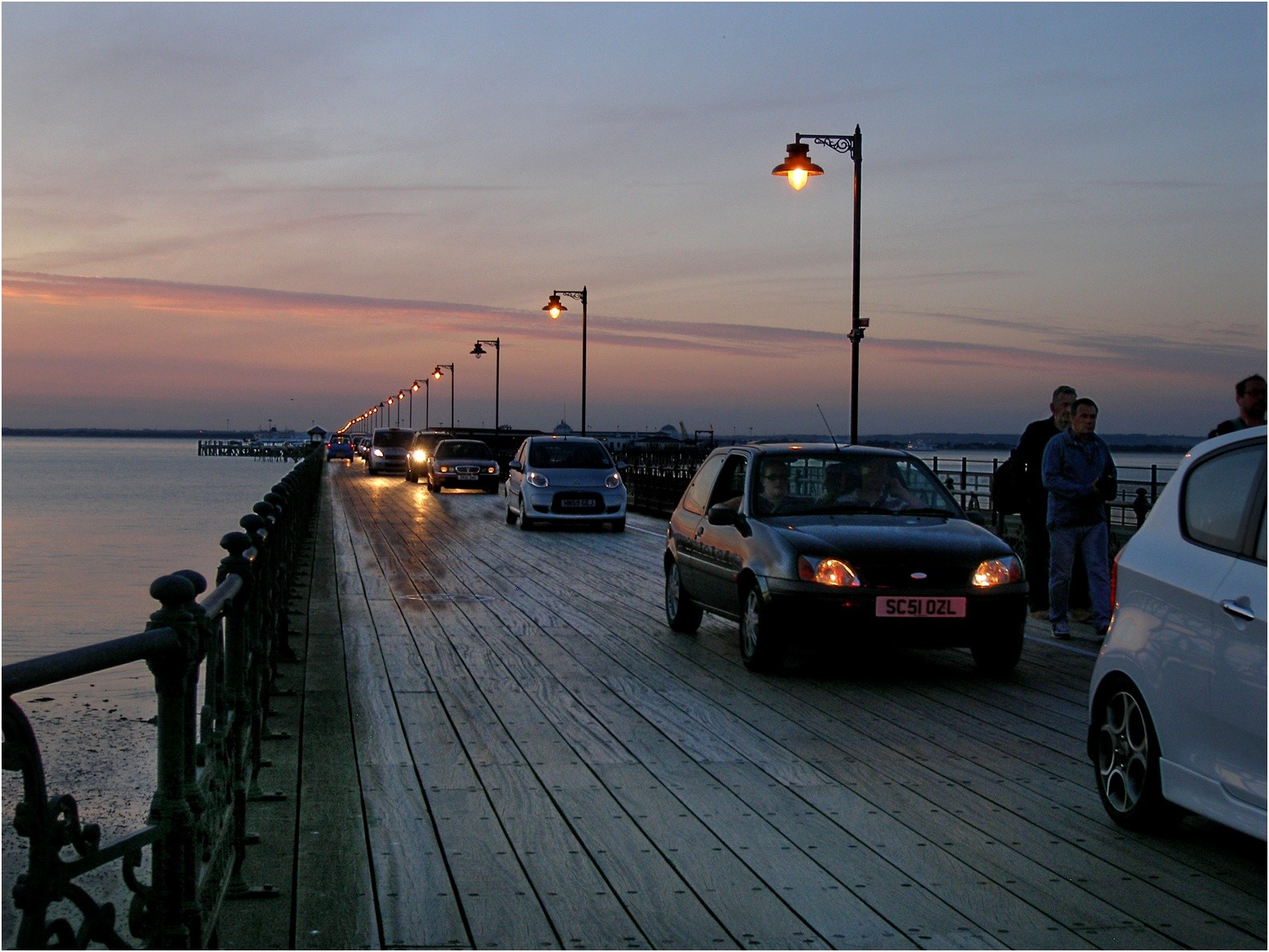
[992, 448, 1029, 515]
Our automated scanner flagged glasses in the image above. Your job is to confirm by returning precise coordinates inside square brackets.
[1243, 390, 1267, 396]
[762, 474, 787, 480]
[1076, 413, 1096, 419]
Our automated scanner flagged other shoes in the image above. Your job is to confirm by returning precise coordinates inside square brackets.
[1070, 609, 1092, 621]
[1031, 608, 1051, 619]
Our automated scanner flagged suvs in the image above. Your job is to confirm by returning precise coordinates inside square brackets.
[403, 430, 456, 484]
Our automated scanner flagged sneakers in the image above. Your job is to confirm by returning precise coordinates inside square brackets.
[1051, 622, 1070, 639]
[1095, 626, 1109, 640]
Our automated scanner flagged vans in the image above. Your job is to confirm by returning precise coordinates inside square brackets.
[367, 427, 416, 478]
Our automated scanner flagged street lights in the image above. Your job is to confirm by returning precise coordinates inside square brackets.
[410, 378, 429, 432]
[432, 362, 455, 432]
[542, 285, 588, 437]
[387, 394, 399, 427]
[768, 123, 872, 446]
[469, 336, 501, 435]
[398, 388, 413, 429]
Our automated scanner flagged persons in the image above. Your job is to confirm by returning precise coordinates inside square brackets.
[1013, 385, 1079, 619]
[720, 460, 801, 515]
[1208, 374, 1267, 439]
[1041, 397, 1117, 640]
[835, 463, 928, 512]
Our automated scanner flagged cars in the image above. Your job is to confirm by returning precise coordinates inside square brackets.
[424, 438, 501, 494]
[662, 437, 1029, 683]
[324, 400, 390, 466]
[503, 434, 628, 532]
[1085, 424, 1267, 852]
